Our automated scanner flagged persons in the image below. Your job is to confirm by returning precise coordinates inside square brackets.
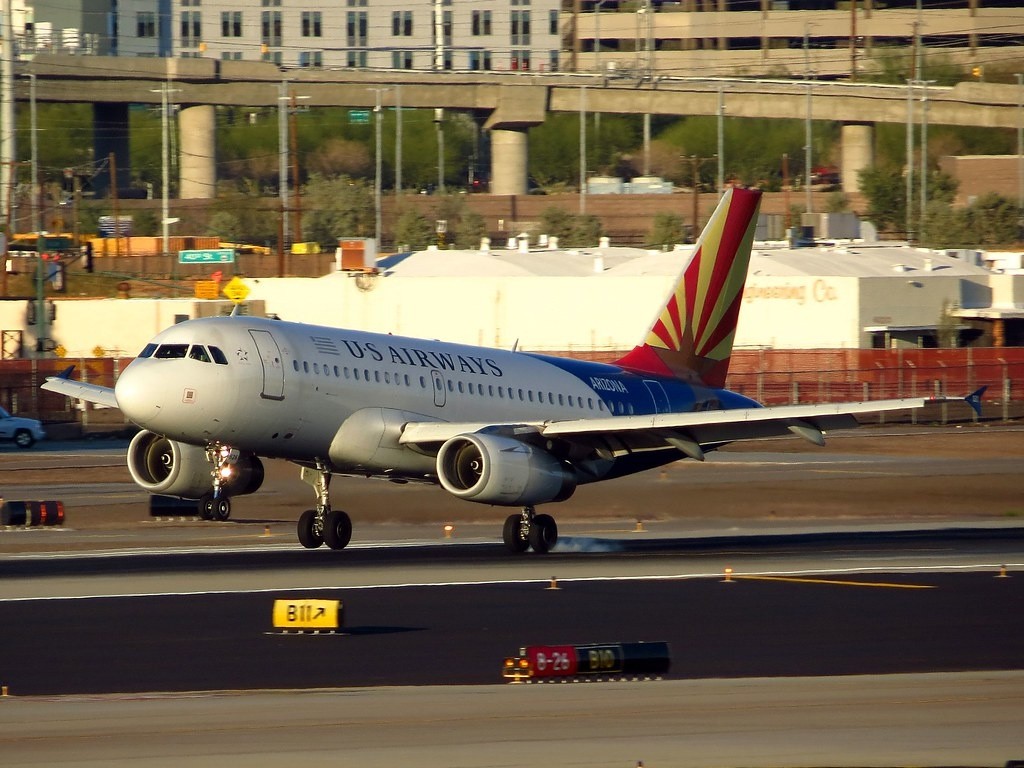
[40, 251, 62, 263]
[528, 175, 538, 194]
[183, 347, 198, 359]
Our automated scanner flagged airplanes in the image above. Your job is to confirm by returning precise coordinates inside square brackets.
[38, 187, 988, 557]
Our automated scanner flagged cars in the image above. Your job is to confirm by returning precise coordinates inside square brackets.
[0, 408, 46, 449]
